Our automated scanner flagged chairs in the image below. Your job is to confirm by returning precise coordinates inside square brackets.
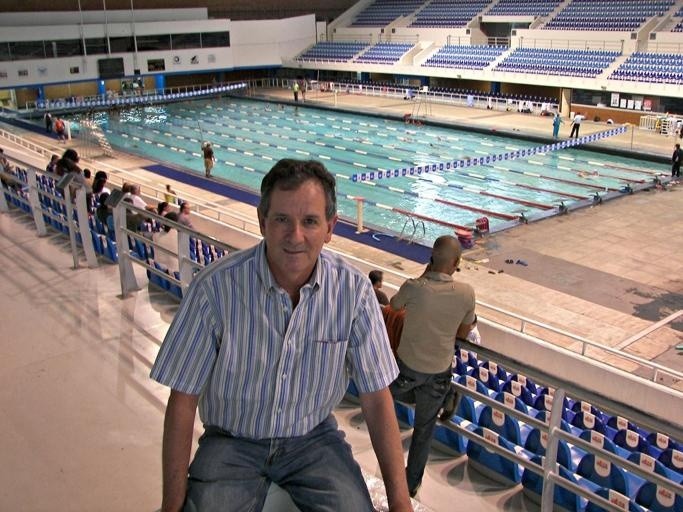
[0, 166, 229, 296]
[345, 348, 683, 512]
[291, 0, 682, 86]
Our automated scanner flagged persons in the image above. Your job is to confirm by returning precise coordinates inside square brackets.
[552, 111, 588, 138]
[292, 77, 308, 102]
[0, 147, 14, 185]
[366, 271, 389, 305]
[201, 141, 215, 177]
[150, 160, 414, 512]
[55, 113, 66, 143]
[383, 236, 481, 498]
[43, 108, 53, 133]
[122, 77, 144, 96]
[45, 149, 197, 241]
[671, 143, 683, 177]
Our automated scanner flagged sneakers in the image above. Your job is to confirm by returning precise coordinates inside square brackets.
[438, 389, 463, 423]
